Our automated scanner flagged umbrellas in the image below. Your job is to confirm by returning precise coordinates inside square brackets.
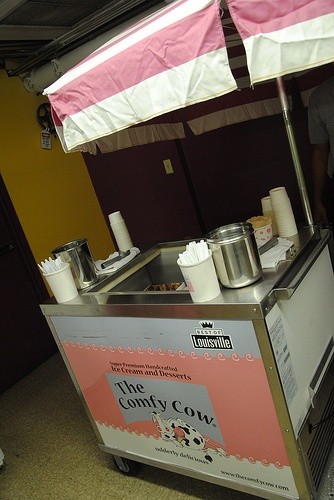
[42, 0, 333, 229]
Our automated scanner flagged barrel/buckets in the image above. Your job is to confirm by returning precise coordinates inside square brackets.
[52, 238, 98, 290]
[207, 222, 262, 289]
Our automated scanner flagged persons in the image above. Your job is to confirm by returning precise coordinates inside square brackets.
[307, 74, 334, 267]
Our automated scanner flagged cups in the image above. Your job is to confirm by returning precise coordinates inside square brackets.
[177, 250, 220, 303]
[108, 212, 133, 252]
[253, 223, 272, 246]
[43, 264, 78, 303]
[262, 187, 297, 237]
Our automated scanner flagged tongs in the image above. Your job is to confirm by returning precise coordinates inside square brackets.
[101, 250, 130, 268]
